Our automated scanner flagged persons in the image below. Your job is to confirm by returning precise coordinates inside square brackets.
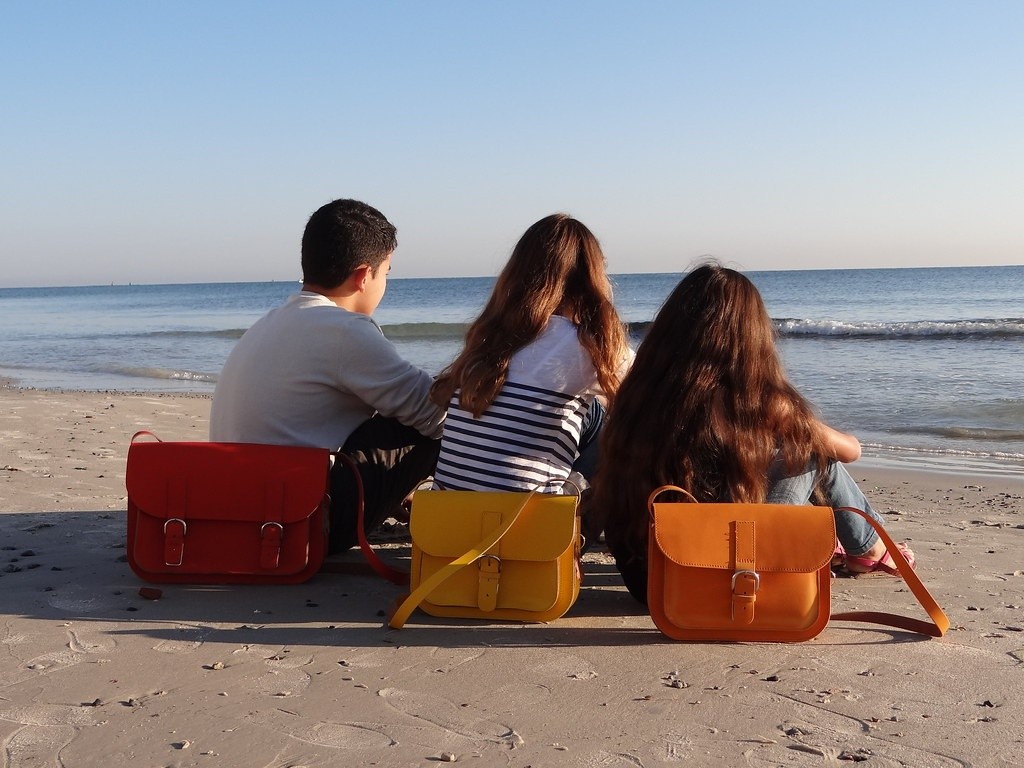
[209, 199, 447, 559]
[430, 214, 635, 558]
[599, 266, 916, 601]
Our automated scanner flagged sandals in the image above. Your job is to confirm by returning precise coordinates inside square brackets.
[836, 540, 916, 578]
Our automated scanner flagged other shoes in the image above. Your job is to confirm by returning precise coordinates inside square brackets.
[395, 490, 416, 526]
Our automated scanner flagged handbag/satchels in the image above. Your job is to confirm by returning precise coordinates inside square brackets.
[387, 476, 585, 633]
[647, 485, 833, 645]
[124, 425, 331, 590]
